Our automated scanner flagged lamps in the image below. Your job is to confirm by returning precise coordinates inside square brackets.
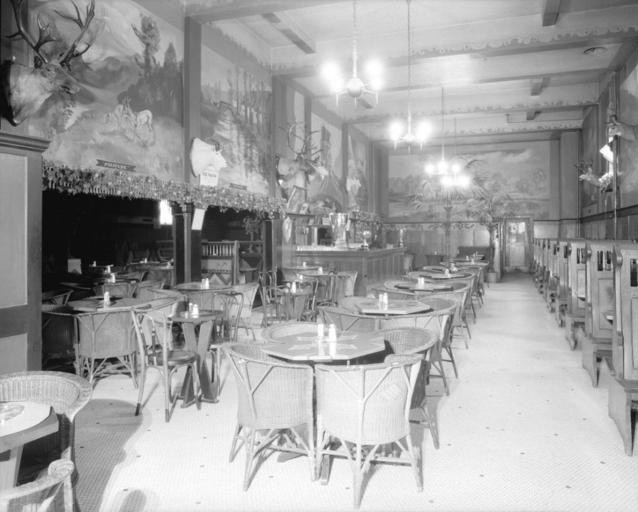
[439, 117, 472, 189]
[387, 2, 435, 153]
[317, 1, 391, 112]
[425, 83, 461, 177]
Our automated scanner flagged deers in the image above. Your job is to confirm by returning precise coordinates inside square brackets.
[604, 114, 638, 143]
[275, 118, 323, 181]
[573, 159, 601, 188]
[0, 0, 97, 130]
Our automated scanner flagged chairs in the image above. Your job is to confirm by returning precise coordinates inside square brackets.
[1, 247, 498, 512]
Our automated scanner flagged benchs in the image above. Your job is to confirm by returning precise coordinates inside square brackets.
[527, 237, 637, 459]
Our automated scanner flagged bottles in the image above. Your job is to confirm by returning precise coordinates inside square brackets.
[105, 266, 116, 284]
[184, 302, 199, 319]
[418, 277, 424, 286]
[201, 277, 209, 290]
[317, 323, 336, 341]
[103, 292, 110, 302]
[379, 292, 388, 305]
[284, 282, 296, 292]
